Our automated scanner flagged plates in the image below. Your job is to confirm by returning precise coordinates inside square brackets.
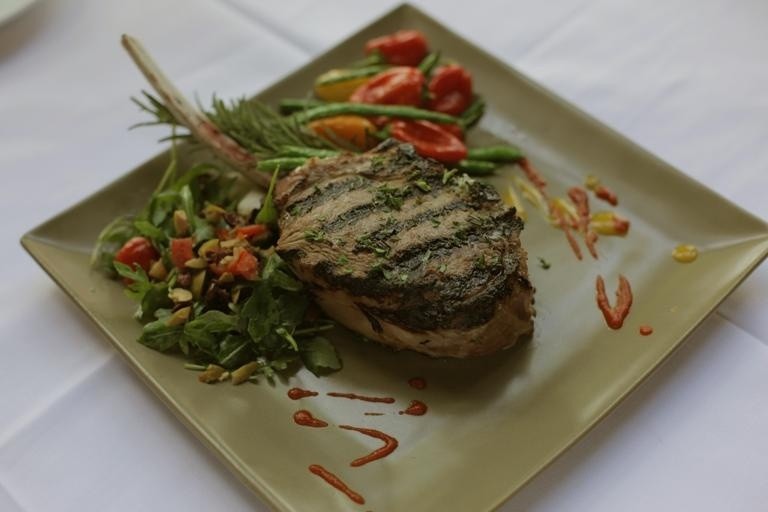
[18, 4, 768, 510]
